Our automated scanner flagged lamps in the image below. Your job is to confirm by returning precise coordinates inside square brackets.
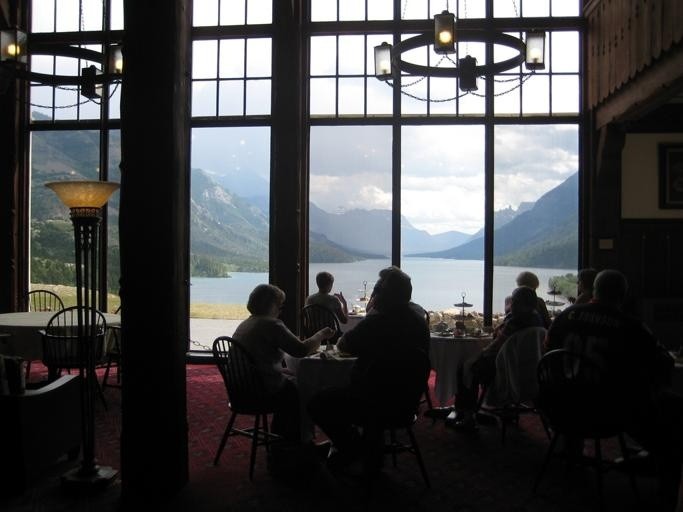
[43, 182, 120, 485]
[0, 24, 123, 101]
[388, 9, 547, 90]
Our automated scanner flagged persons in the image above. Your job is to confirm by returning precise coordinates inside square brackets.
[543, 268, 681, 461]
[366, 292, 427, 317]
[304, 273, 349, 346]
[304, 266, 431, 472]
[504, 271, 552, 331]
[227, 283, 337, 467]
[577, 270, 598, 302]
[443, 285, 543, 429]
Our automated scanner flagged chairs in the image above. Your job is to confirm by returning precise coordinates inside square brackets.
[524, 347, 642, 511]
[409, 302, 434, 414]
[16, 290, 65, 327]
[472, 325, 551, 442]
[37, 306, 108, 410]
[103, 322, 122, 391]
[213, 329, 306, 482]
[371, 350, 432, 489]
[0, 355, 96, 499]
[299, 304, 343, 345]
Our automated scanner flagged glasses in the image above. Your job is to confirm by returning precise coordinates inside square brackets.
[274, 305, 285, 313]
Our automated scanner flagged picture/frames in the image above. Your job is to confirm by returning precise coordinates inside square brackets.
[614, 129, 683, 225]
